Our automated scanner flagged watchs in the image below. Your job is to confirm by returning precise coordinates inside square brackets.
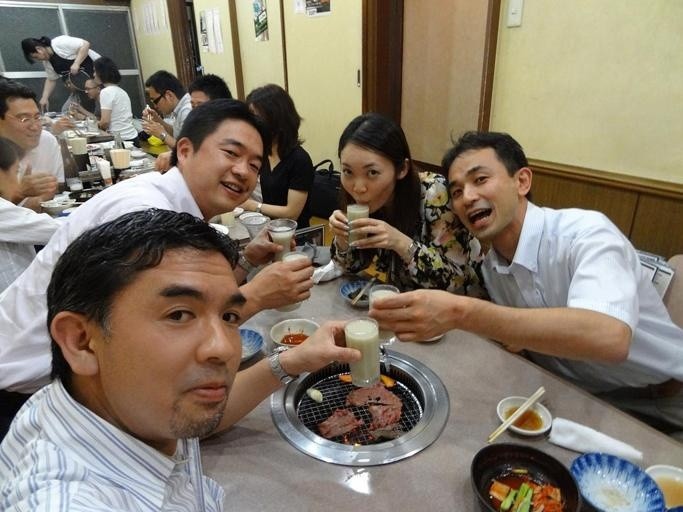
[268, 344, 300, 386]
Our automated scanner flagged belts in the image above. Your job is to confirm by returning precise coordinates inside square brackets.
[604, 379, 682, 404]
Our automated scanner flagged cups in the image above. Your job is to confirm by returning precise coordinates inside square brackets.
[275, 249, 308, 313]
[68, 179, 82, 190]
[370, 284, 401, 342]
[113, 166, 131, 181]
[86, 114, 98, 131]
[71, 152, 91, 172]
[241, 214, 269, 247]
[343, 317, 382, 387]
[267, 217, 298, 258]
[220, 212, 236, 229]
[346, 204, 370, 250]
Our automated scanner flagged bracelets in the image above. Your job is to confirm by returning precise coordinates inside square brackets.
[257, 203, 262, 213]
[401, 241, 418, 264]
[237, 256, 253, 273]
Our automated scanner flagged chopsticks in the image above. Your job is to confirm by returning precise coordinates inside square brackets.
[351, 275, 378, 305]
[70, 126, 85, 137]
[483, 385, 546, 444]
[145, 103, 151, 122]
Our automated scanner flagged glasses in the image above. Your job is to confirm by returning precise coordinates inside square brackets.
[5, 114, 41, 126]
[149, 94, 163, 106]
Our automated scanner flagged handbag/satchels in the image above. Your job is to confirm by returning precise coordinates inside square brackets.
[307, 159, 344, 220]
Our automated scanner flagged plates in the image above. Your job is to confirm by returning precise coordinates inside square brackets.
[130, 150, 146, 158]
[233, 206, 244, 218]
[85, 143, 99, 152]
[239, 327, 265, 364]
[111, 141, 133, 149]
[208, 222, 229, 236]
[239, 211, 261, 219]
[570, 453, 665, 512]
[69, 188, 103, 201]
[340, 280, 371, 307]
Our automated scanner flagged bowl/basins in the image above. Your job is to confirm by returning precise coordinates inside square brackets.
[470, 443, 581, 512]
[270, 317, 320, 347]
[41, 197, 75, 215]
[494, 395, 552, 435]
[641, 463, 682, 508]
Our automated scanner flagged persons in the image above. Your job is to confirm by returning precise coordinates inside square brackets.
[68, 55, 234, 172]
[0, 137, 65, 295]
[328, 113, 489, 301]
[240, 83, 314, 229]
[0, 79, 65, 212]
[20, 33, 102, 114]
[0, 207, 364, 512]
[0, 98, 314, 434]
[368, 129, 683, 447]
